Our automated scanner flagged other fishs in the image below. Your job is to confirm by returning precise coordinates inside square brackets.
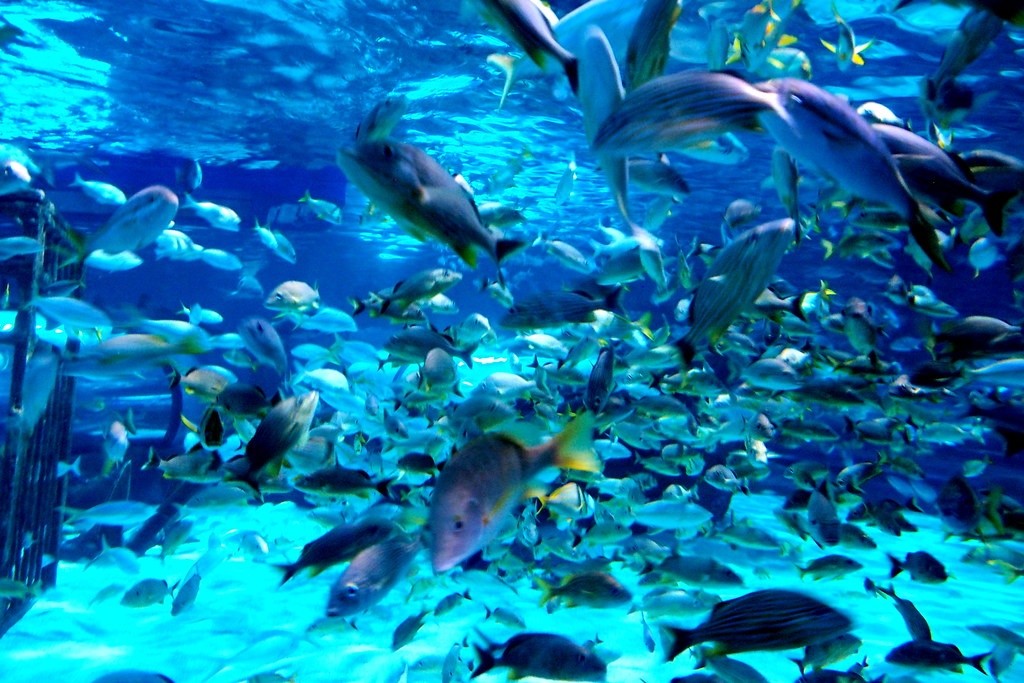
[0, 0, 1024, 683]
